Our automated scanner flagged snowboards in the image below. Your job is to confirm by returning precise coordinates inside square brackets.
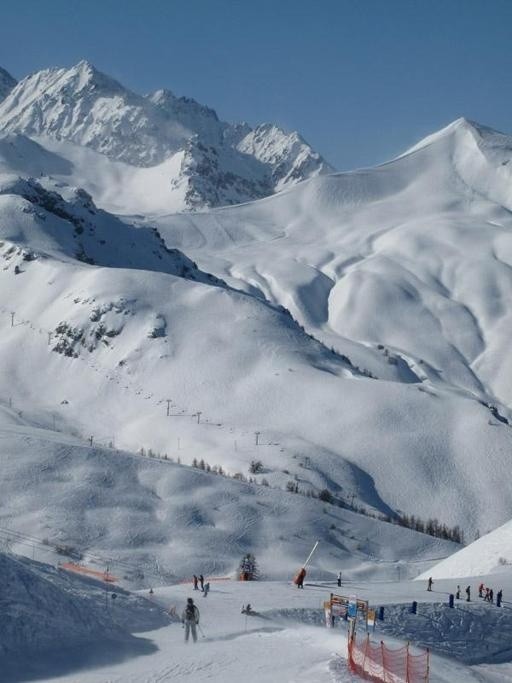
[323, 594, 377, 634]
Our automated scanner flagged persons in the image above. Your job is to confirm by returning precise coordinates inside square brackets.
[489, 589, 493, 603]
[337, 571, 341, 586]
[427, 576, 432, 590]
[496, 589, 502, 607]
[193, 574, 199, 589]
[198, 574, 205, 591]
[202, 581, 209, 596]
[478, 583, 484, 597]
[457, 585, 461, 593]
[465, 585, 470, 600]
[182, 597, 200, 643]
[483, 587, 490, 602]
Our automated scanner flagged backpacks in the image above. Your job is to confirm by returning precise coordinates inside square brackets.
[185, 605, 195, 620]
